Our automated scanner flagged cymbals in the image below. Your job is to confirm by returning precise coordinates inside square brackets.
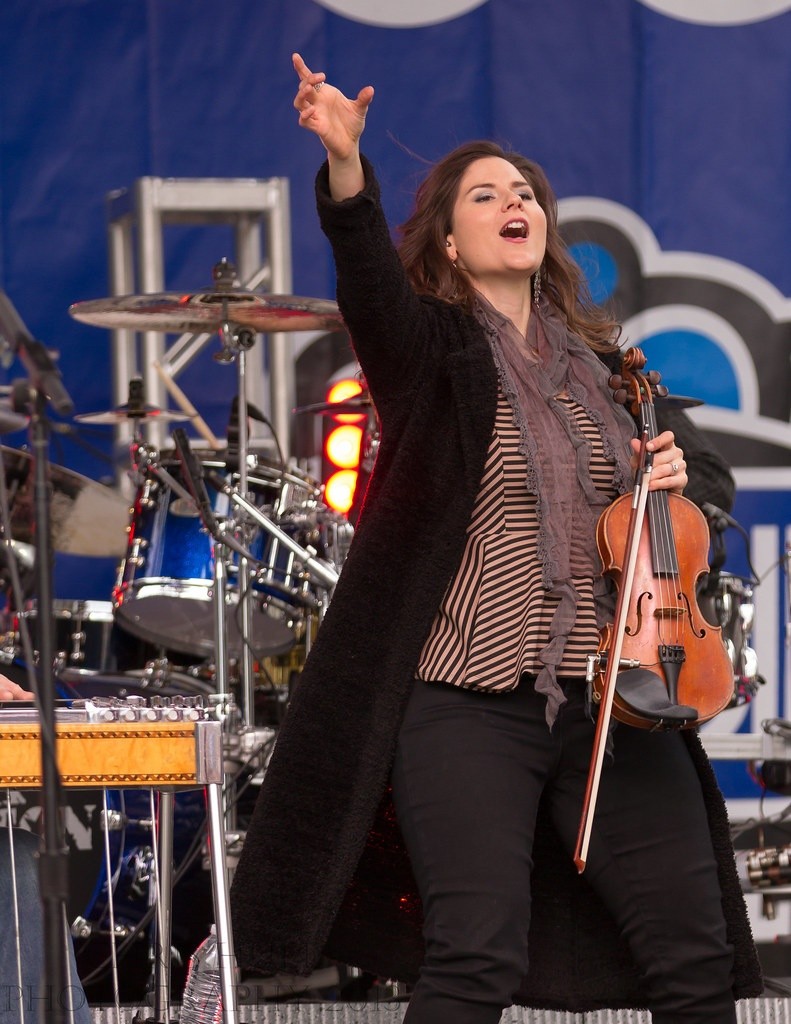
[0, 444, 131, 559]
[67, 290, 345, 336]
[72, 404, 193, 425]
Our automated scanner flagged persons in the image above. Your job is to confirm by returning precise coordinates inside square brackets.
[0, 668, 100, 1022]
[228, 54, 764, 1023]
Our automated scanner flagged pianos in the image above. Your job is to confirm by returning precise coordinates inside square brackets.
[1, 691, 242, 1023]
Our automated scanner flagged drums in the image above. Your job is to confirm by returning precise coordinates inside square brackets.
[1, 648, 213, 992]
[108, 446, 326, 660]
[14, 595, 209, 681]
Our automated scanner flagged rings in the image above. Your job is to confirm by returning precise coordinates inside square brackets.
[314, 81, 324, 92]
[670, 462, 678, 476]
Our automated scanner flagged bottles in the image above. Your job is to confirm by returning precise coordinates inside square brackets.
[179, 924, 223, 1024]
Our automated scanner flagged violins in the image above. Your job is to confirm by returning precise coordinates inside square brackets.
[590, 343, 739, 736]
